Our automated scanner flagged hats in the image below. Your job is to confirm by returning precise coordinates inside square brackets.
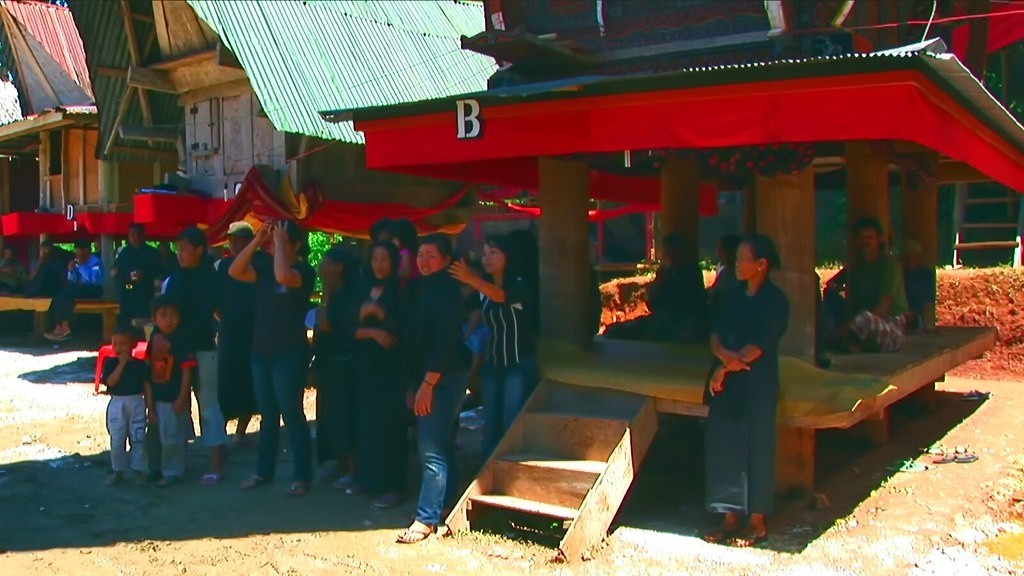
[226, 222, 254, 237]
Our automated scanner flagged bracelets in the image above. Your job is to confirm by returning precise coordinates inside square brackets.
[423, 379, 435, 387]
[274, 234, 285, 241]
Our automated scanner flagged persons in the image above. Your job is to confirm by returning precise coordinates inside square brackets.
[603, 233, 741, 343]
[101, 323, 157, 487]
[824, 217, 910, 354]
[228, 219, 316, 499]
[144, 297, 197, 492]
[397, 233, 469, 545]
[897, 238, 939, 336]
[701, 234, 791, 546]
[0, 219, 534, 509]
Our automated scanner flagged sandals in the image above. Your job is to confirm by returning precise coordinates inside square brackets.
[397, 520, 437, 543]
[443, 511, 449, 523]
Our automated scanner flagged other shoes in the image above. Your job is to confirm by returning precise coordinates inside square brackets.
[331, 475, 354, 488]
[159, 476, 181, 487]
[105, 475, 121, 485]
[918, 440, 979, 463]
[962, 390, 992, 400]
[134, 477, 146, 486]
[44, 327, 74, 342]
[374, 495, 408, 508]
[234, 437, 258, 447]
[314, 470, 335, 481]
[345, 485, 367, 495]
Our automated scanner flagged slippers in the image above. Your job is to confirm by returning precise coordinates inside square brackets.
[198, 472, 226, 485]
[703, 529, 736, 542]
[242, 477, 273, 489]
[727, 530, 769, 546]
[287, 483, 311, 495]
[885, 456, 928, 472]
